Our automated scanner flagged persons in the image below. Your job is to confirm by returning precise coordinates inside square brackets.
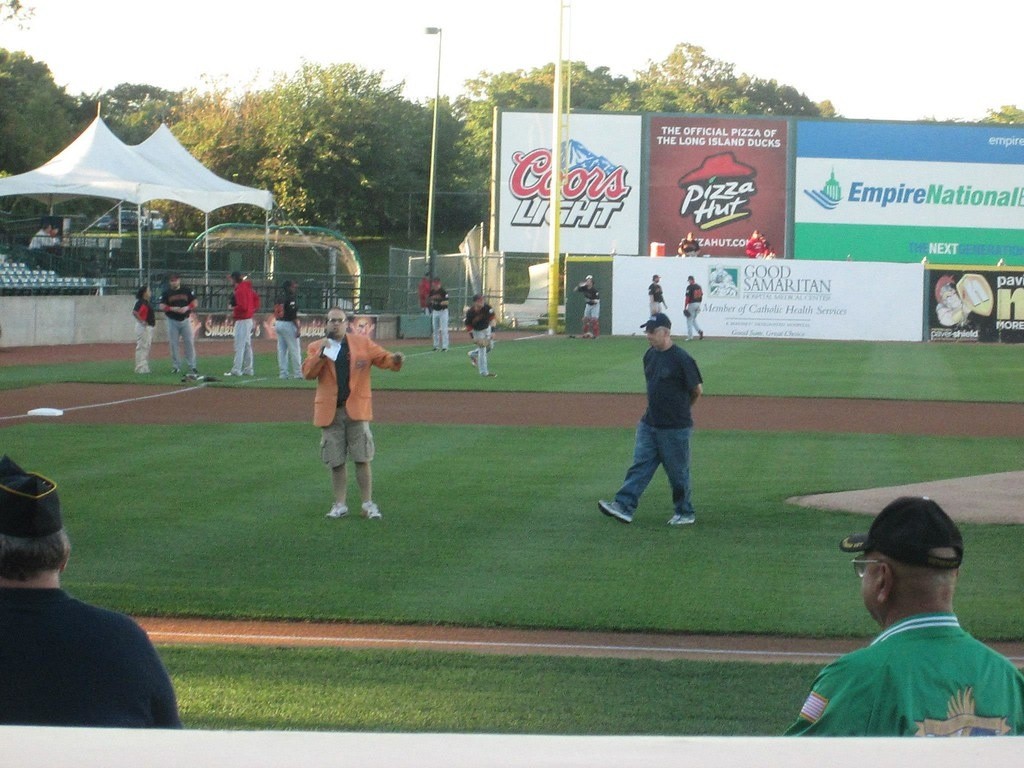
[156, 272, 200, 373]
[684, 276, 703, 341]
[274, 277, 303, 378]
[27, 223, 52, 250]
[45, 224, 62, 246]
[225, 271, 259, 375]
[419, 273, 432, 313]
[465, 294, 497, 376]
[425, 277, 449, 352]
[678, 232, 701, 257]
[746, 230, 776, 258]
[599, 313, 704, 524]
[132, 285, 155, 374]
[573, 277, 600, 339]
[648, 275, 667, 315]
[303, 307, 404, 519]
[782, 494, 1024, 738]
[0, 454, 182, 730]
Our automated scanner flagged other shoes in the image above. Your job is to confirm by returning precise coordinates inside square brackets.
[224, 371, 242, 376]
[434, 348, 439, 352]
[699, 331, 703, 339]
[487, 372, 498, 377]
[191, 367, 199, 374]
[171, 368, 180, 373]
[685, 338, 693, 341]
[467, 351, 478, 366]
[442, 349, 447, 352]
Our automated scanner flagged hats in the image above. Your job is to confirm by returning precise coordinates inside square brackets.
[841, 495, 963, 569]
[687, 275, 693, 280]
[473, 294, 486, 302]
[433, 278, 441, 285]
[0, 454, 61, 536]
[228, 271, 242, 281]
[653, 275, 661, 279]
[169, 272, 180, 281]
[641, 313, 671, 329]
[283, 281, 296, 288]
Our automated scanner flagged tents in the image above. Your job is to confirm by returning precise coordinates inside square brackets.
[0, 117, 273, 285]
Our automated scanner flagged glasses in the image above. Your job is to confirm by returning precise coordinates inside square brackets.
[645, 328, 664, 333]
[325, 318, 349, 324]
[851, 553, 883, 578]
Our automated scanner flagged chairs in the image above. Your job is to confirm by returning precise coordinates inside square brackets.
[0, 254, 118, 296]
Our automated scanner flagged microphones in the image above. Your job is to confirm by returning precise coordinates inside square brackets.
[319, 331, 334, 358]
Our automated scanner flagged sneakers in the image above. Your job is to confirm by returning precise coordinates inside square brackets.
[598, 499, 633, 523]
[361, 500, 381, 518]
[667, 513, 695, 524]
[326, 504, 348, 518]
